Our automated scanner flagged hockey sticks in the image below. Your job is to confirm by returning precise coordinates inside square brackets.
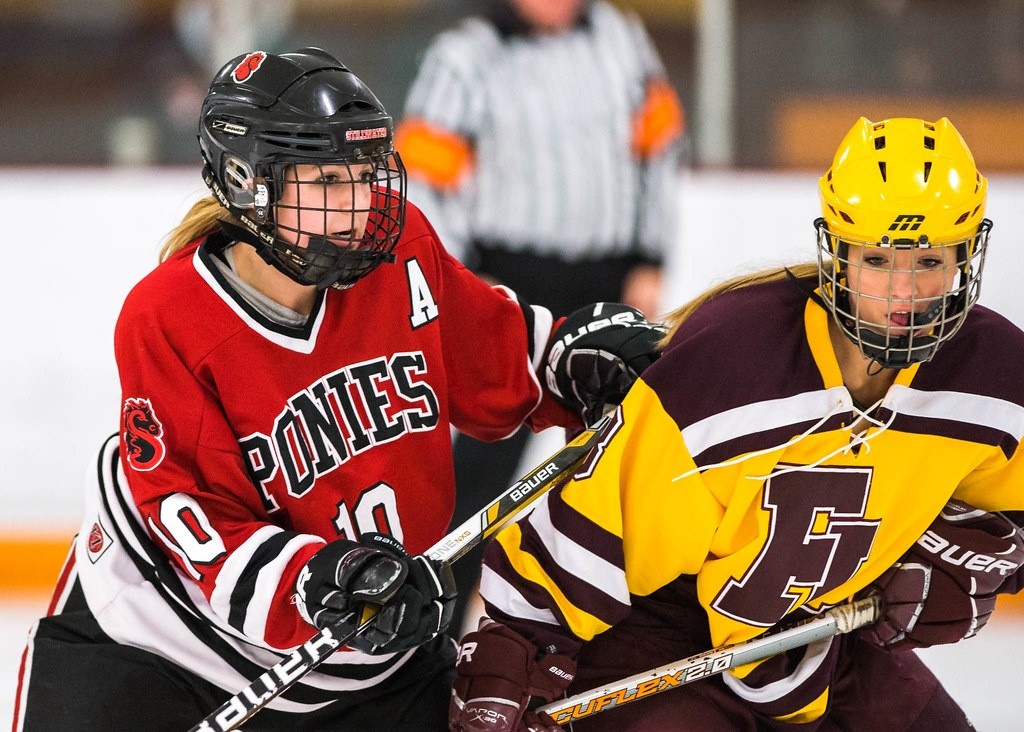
[532, 594, 879, 729]
[183, 405, 615, 732]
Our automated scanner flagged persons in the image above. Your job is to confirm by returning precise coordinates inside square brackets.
[448, 114, 1022, 732]
[10, 45, 666, 731]
[388, 1, 686, 640]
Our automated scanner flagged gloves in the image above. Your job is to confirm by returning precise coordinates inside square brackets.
[539, 301, 674, 432]
[289, 531, 457, 656]
[856, 498, 1024, 654]
[449, 616, 578, 732]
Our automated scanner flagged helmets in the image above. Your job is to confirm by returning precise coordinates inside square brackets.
[814, 116, 994, 370]
[196, 46, 407, 290]
